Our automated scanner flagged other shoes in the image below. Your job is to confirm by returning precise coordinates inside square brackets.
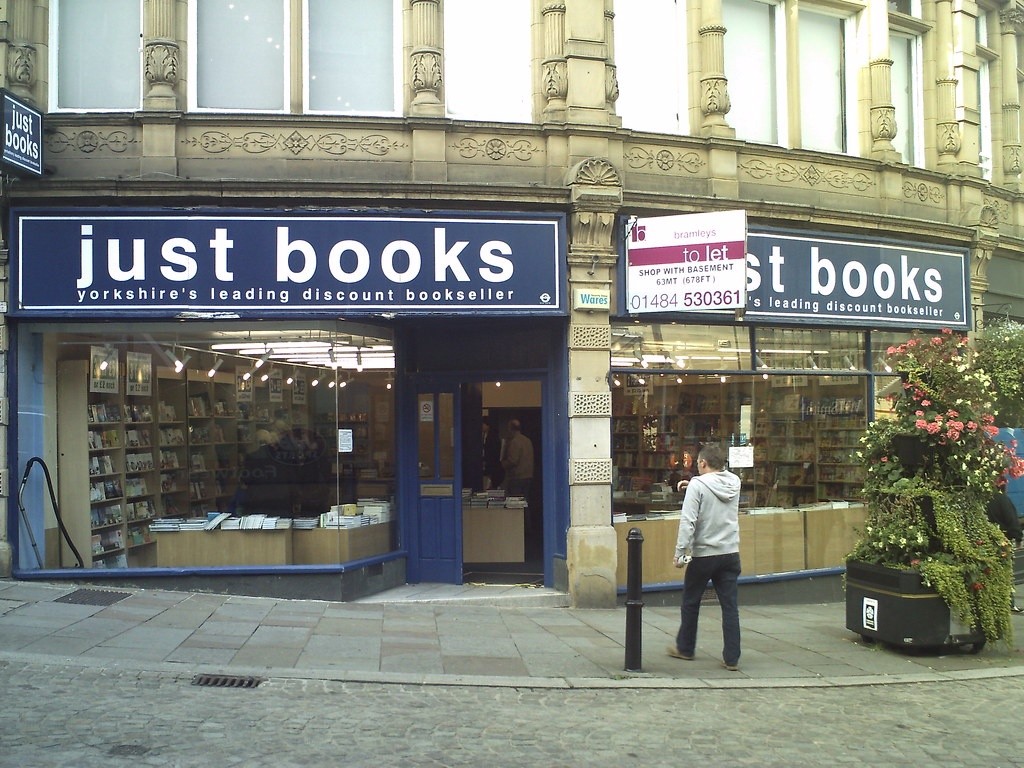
[721, 659, 737, 670]
[667, 644, 694, 659]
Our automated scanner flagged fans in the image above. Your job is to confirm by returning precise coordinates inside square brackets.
[220, 332, 268, 343]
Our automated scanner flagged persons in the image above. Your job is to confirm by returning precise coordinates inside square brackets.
[240, 422, 329, 518]
[482, 415, 534, 537]
[988, 478, 1024, 614]
[668, 444, 741, 671]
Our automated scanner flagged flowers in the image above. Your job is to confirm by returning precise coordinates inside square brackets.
[842, 316, 1024, 649]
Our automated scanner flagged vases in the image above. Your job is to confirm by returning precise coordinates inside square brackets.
[846, 555, 986, 648]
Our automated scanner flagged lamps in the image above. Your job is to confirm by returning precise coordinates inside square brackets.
[174, 349, 192, 373]
[165, 345, 182, 367]
[876, 356, 892, 373]
[208, 354, 223, 376]
[807, 357, 818, 369]
[610, 350, 726, 385]
[261, 369, 279, 382]
[755, 358, 766, 368]
[286, 366, 300, 383]
[254, 349, 274, 368]
[340, 374, 355, 387]
[324, 354, 339, 370]
[312, 369, 326, 386]
[841, 355, 855, 370]
[328, 372, 342, 388]
[355, 355, 364, 372]
[100, 345, 116, 372]
[241, 361, 260, 379]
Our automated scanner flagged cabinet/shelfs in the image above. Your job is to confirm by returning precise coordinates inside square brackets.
[612, 383, 869, 508]
[59, 360, 309, 568]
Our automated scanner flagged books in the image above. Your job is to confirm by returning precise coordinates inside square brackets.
[462, 487, 528, 509]
[611, 391, 867, 524]
[87, 395, 157, 569]
[318, 462, 397, 529]
[237, 401, 370, 466]
[157, 393, 244, 519]
[672, 554, 692, 568]
[148, 513, 319, 532]
